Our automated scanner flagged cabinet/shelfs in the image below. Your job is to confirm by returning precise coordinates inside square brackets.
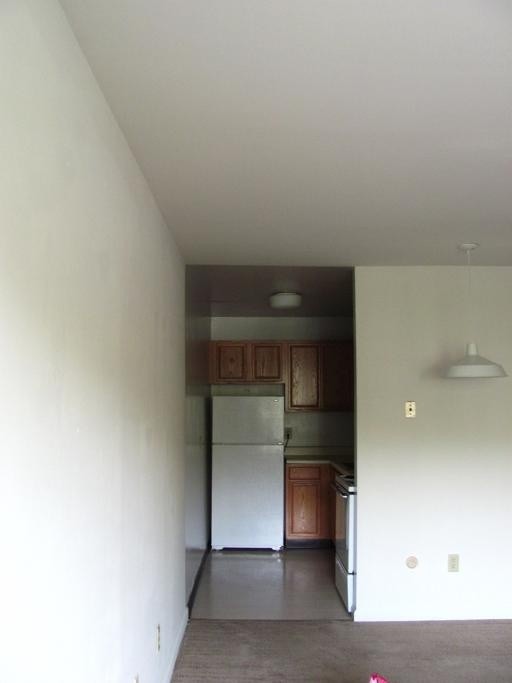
[212, 340, 285, 385]
[285, 340, 354, 413]
[285, 463, 331, 538]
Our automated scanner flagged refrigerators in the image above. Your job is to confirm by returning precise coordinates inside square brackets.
[207, 385, 289, 554]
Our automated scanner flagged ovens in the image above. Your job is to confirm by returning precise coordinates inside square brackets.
[330, 479, 355, 606]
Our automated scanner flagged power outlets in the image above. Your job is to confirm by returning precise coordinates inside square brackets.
[284, 428, 293, 439]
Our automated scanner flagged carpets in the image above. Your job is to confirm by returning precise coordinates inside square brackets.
[170, 618, 512, 683]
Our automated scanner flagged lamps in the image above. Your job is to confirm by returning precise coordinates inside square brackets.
[270, 292, 302, 310]
[445, 243, 507, 379]
